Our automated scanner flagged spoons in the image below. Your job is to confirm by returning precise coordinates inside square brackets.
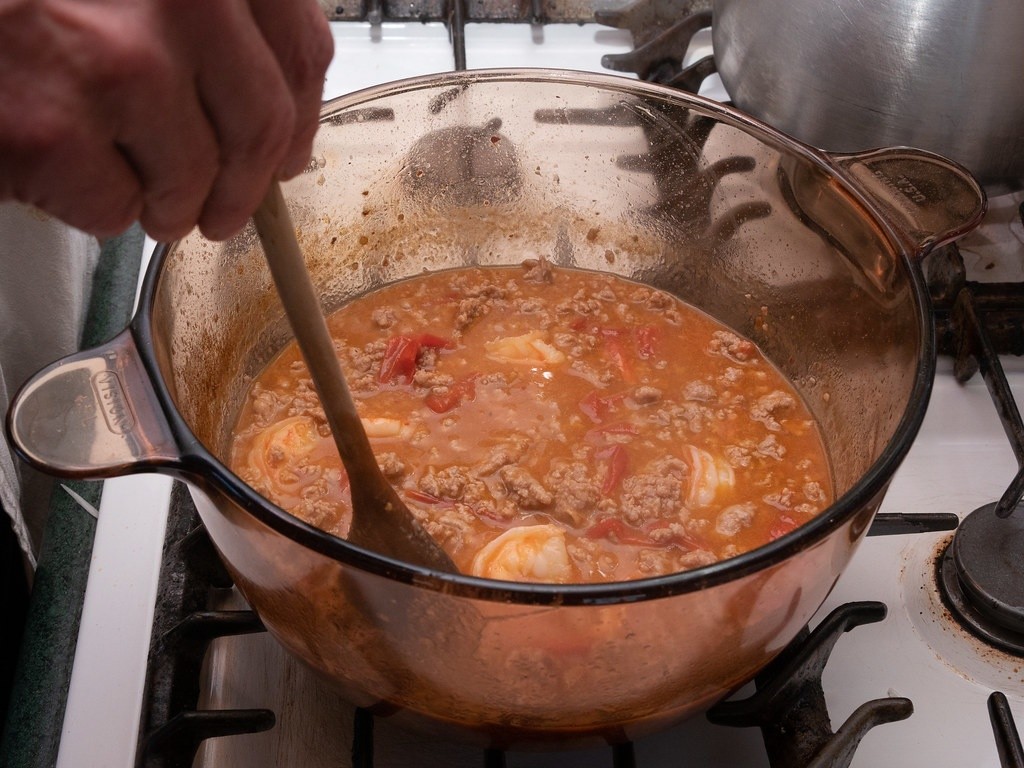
[250, 171, 460, 626]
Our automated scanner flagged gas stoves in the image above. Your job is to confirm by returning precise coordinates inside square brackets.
[2, 5, 1022, 768]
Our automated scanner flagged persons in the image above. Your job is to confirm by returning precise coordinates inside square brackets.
[1, 0, 336, 712]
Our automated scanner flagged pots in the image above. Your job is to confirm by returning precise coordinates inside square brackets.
[710, 1, 1024, 187]
[3, 66, 988, 733]
